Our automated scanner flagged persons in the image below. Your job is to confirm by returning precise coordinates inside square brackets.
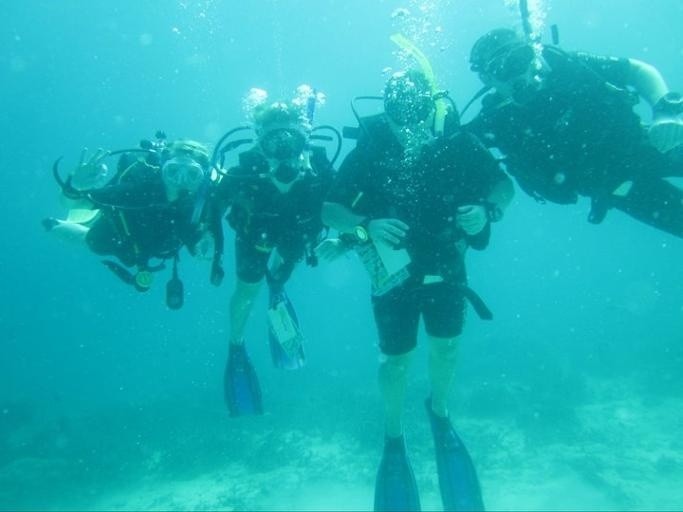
[322, 70, 514, 511]
[46, 140, 225, 310]
[214, 92, 342, 415]
[461, 27, 683, 239]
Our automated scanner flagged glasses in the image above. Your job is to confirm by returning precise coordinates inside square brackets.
[266, 130, 305, 154]
[385, 95, 434, 124]
[163, 159, 203, 187]
[488, 45, 536, 79]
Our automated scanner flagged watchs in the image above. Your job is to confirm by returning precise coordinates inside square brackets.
[652, 91, 682, 115]
[354, 217, 371, 244]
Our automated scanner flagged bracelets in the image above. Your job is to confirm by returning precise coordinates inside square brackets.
[478, 201, 503, 223]
